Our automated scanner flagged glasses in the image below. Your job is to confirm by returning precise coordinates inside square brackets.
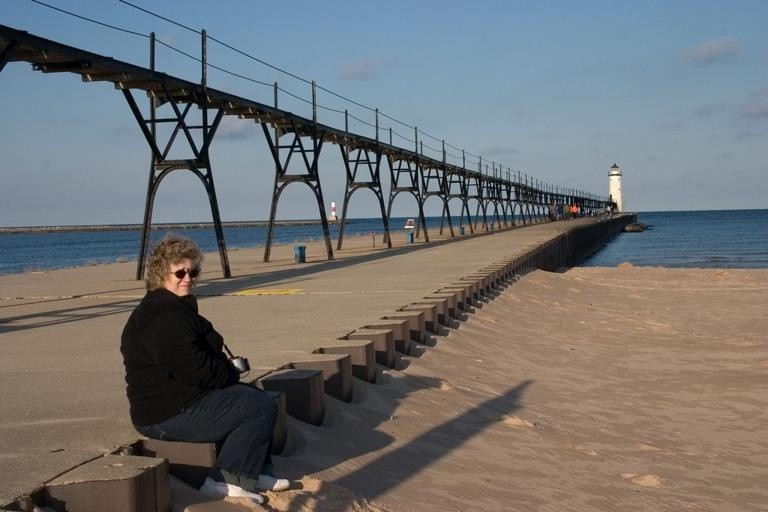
[168, 268, 199, 278]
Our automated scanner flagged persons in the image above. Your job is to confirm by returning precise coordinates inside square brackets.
[117, 234, 291, 504]
[548, 203, 585, 221]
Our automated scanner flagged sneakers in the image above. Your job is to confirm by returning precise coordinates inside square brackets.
[199, 477, 264, 504]
[238, 474, 290, 492]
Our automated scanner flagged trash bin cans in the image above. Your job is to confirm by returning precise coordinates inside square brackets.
[460, 226, 464, 235]
[294, 246, 305, 262]
[490, 223, 494, 230]
[407, 232, 414, 243]
